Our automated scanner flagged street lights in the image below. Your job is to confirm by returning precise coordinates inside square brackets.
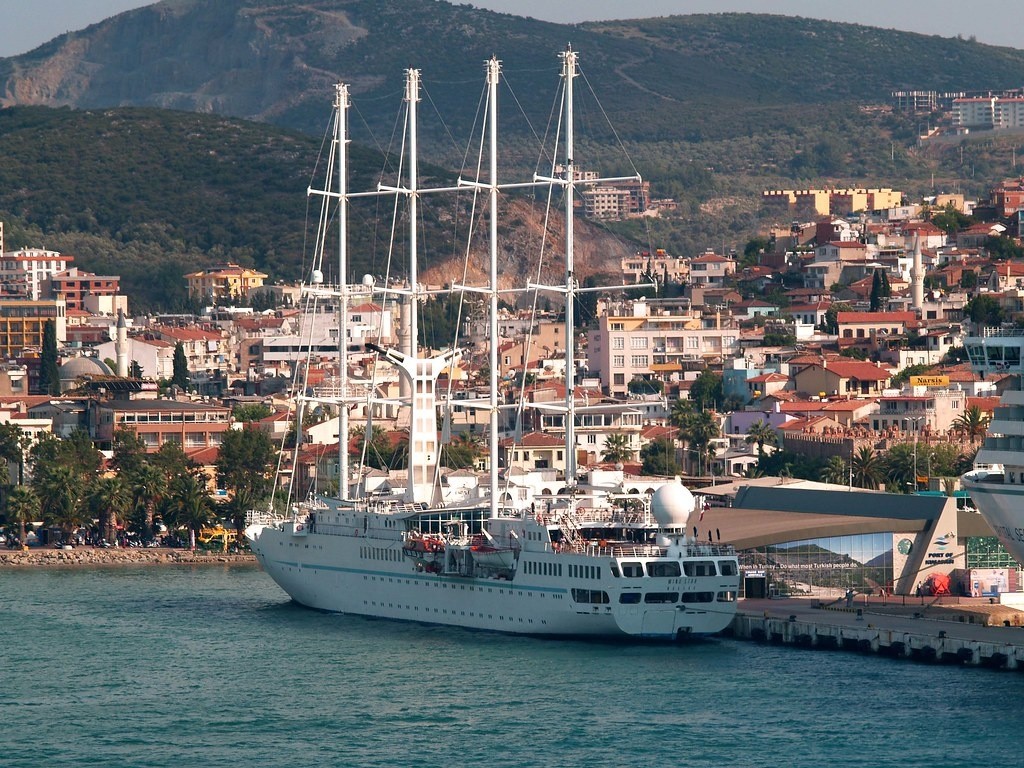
[905, 416, 925, 491]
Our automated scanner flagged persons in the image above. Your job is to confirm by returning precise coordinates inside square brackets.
[75, 531, 186, 548]
[916, 581, 924, 597]
[881, 587, 886, 603]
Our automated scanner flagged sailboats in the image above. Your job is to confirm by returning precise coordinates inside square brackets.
[243, 50, 740, 643]
